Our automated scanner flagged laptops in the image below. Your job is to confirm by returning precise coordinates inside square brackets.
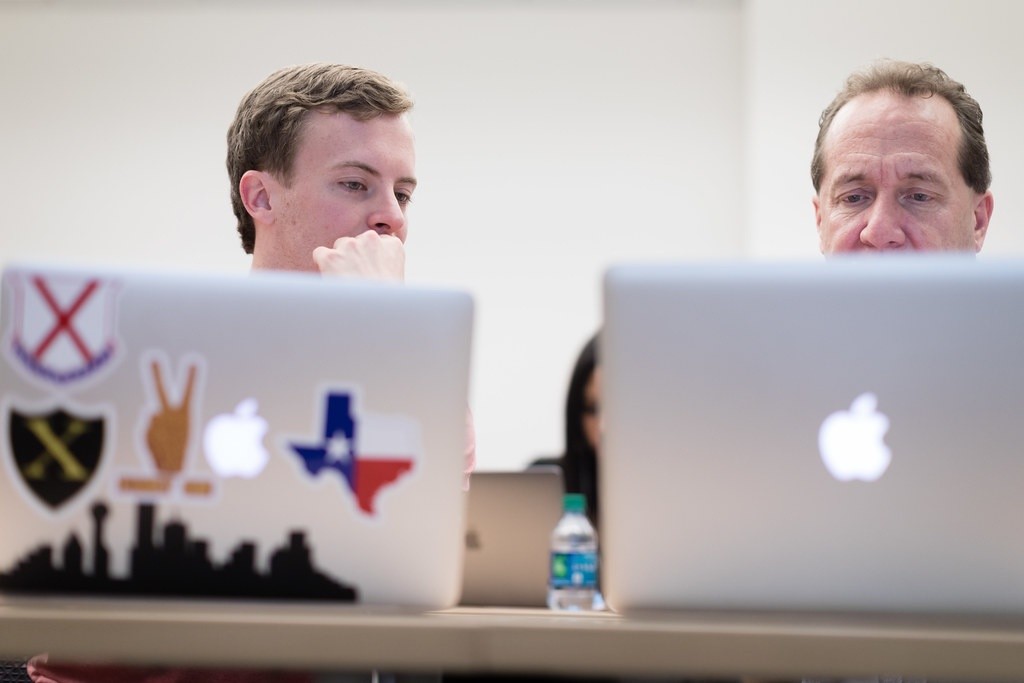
[593, 251, 1024, 620]
[0, 264, 476, 604]
[460, 466, 562, 608]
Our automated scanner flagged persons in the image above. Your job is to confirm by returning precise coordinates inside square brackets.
[525, 330, 603, 612]
[226, 63, 422, 284]
[809, 58, 994, 258]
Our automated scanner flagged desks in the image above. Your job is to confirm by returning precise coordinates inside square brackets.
[0, 597, 1024, 683]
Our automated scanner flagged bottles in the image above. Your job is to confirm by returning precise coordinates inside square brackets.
[546, 493, 598, 612]
